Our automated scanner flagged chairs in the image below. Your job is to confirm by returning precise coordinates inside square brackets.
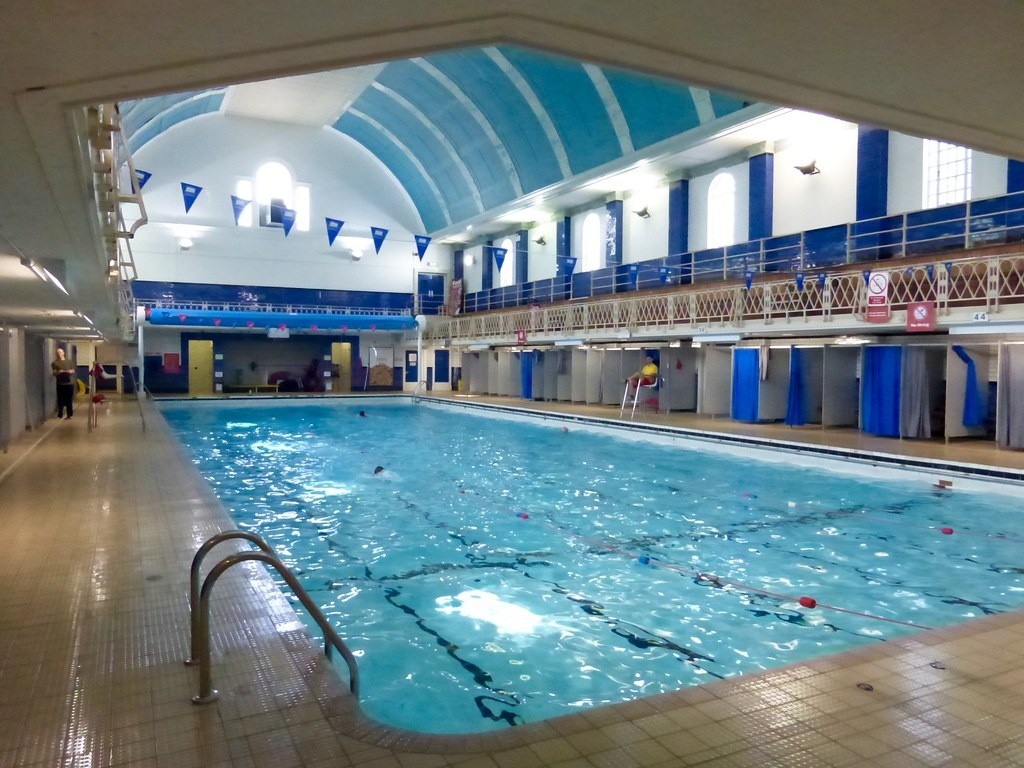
[88, 359, 145, 433]
[620, 374, 663, 420]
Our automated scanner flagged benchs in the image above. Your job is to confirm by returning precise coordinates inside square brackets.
[229, 384, 279, 393]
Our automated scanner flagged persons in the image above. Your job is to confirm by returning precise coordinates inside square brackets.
[51, 347, 75, 419]
[627, 355, 658, 400]
